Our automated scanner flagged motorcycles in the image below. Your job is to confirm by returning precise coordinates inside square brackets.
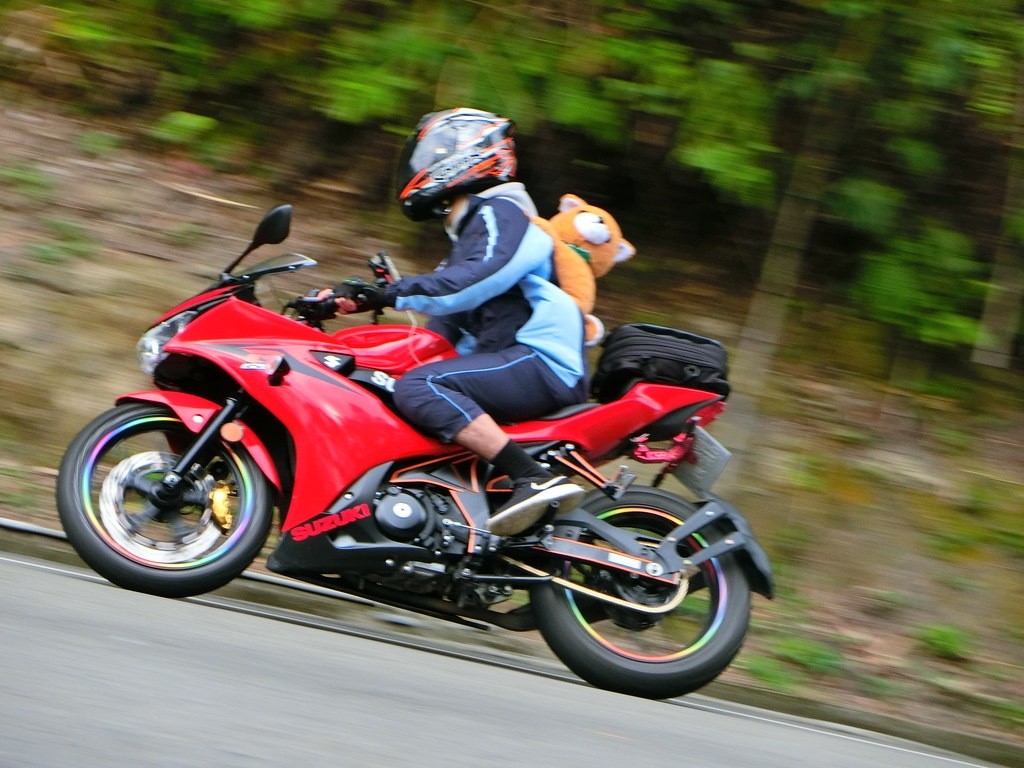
[55, 203, 780, 700]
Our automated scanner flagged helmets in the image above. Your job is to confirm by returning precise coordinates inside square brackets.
[395, 106, 518, 222]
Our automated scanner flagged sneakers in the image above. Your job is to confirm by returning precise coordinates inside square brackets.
[485, 475, 587, 536]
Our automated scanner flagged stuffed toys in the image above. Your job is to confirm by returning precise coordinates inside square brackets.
[535, 192, 634, 351]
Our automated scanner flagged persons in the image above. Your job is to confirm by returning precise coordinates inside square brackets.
[278, 105, 588, 537]
